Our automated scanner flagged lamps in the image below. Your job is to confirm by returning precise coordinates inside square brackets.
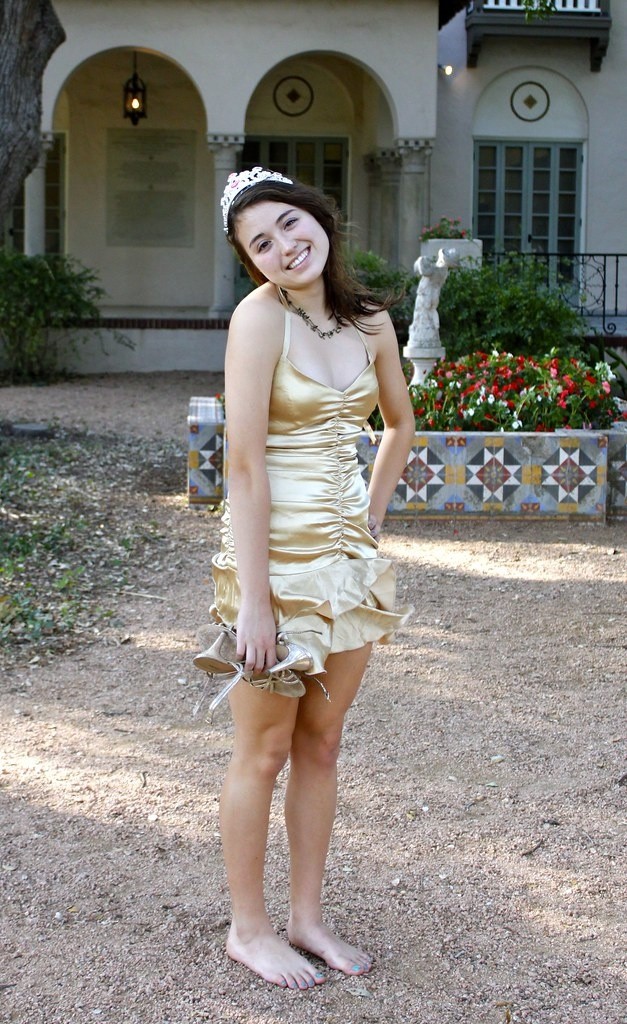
[123, 50, 148, 126]
[438, 62, 454, 76]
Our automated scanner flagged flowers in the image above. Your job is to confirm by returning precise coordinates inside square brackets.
[419, 210, 478, 243]
[215, 342, 627, 433]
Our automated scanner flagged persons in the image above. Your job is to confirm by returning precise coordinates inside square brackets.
[208, 164, 415, 990]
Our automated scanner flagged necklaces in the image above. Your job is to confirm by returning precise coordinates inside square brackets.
[277, 289, 341, 341]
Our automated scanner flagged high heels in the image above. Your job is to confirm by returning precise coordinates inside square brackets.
[192, 632, 306, 723]
[194, 622, 331, 704]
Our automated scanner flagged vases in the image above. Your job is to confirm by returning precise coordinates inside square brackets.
[420, 238, 482, 272]
[186, 415, 627, 529]
